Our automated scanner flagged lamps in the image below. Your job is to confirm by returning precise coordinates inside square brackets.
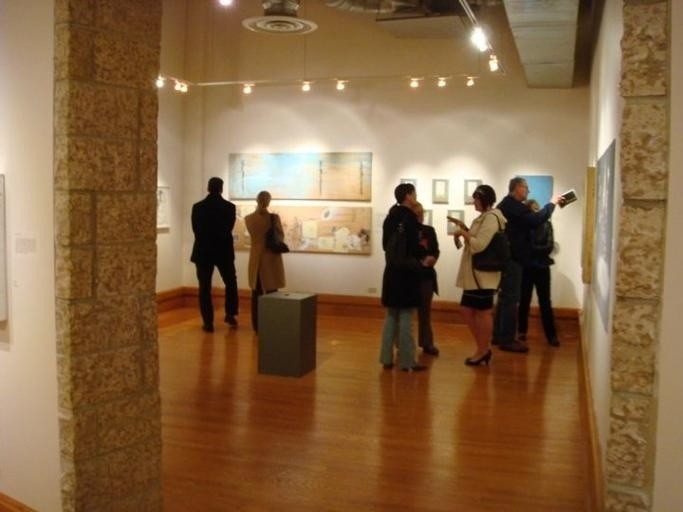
[199, 70, 484, 95]
[153, 74, 195, 96]
[465, 20, 502, 76]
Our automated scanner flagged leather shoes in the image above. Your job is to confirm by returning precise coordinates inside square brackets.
[518, 333, 526, 341]
[548, 338, 560, 346]
[224, 314, 237, 325]
[499, 342, 528, 353]
[202, 325, 214, 332]
[383, 362, 394, 369]
[423, 346, 439, 355]
[404, 363, 427, 371]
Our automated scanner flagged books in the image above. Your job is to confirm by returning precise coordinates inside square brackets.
[560, 190, 577, 208]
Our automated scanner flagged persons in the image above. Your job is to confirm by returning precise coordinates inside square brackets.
[396, 203, 440, 356]
[245, 192, 289, 335]
[379, 183, 428, 371]
[190, 177, 238, 333]
[517, 199, 559, 345]
[447, 185, 507, 365]
[491, 177, 565, 351]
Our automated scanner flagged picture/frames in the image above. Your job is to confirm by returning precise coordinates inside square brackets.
[446, 209, 466, 237]
[399, 179, 417, 188]
[430, 178, 448, 205]
[155, 183, 175, 233]
[463, 178, 482, 206]
[422, 209, 432, 227]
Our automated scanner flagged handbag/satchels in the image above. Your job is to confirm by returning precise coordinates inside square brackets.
[265, 213, 289, 255]
[476, 213, 512, 271]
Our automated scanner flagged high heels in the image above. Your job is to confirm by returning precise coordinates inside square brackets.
[465, 350, 492, 366]
